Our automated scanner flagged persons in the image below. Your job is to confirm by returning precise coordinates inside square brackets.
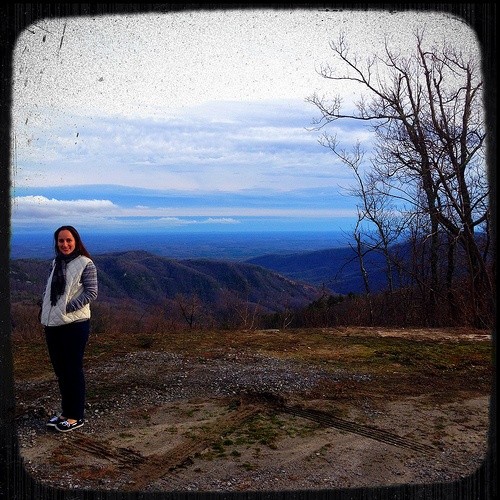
[37, 225, 98, 432]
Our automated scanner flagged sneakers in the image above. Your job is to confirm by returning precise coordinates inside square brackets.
[46, 415, 85, 432]
